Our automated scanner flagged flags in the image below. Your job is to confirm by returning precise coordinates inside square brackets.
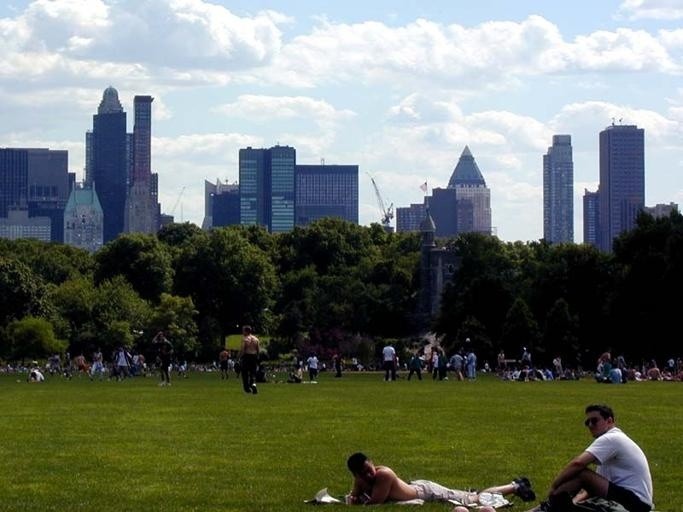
[420, 181, 426, 193]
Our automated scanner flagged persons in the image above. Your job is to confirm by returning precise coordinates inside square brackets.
[453, 505, 496, 512]
[353, 354, 366, 371]
[483, 347, 585, 382]
[596, 348, 683, 383]
[345, 453, 535, 504]
[382, 343, 397, 380]
[257, 347, 342, 384]
[237, 326, 260, 392]
[153, 329, 174, 387]
[1, 347, 240, 383]
[528, 404, 656, 512]
[407, 349, 476, 379]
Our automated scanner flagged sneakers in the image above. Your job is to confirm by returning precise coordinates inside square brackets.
[244, 382, 257, 395]
[157, 381, 171, 387]
[511, 475, 536, 502]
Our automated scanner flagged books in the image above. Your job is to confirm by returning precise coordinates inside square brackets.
[303, 488, 341, 504]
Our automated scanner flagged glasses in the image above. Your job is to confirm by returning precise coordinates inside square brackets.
[583, 415, 603, 426]
